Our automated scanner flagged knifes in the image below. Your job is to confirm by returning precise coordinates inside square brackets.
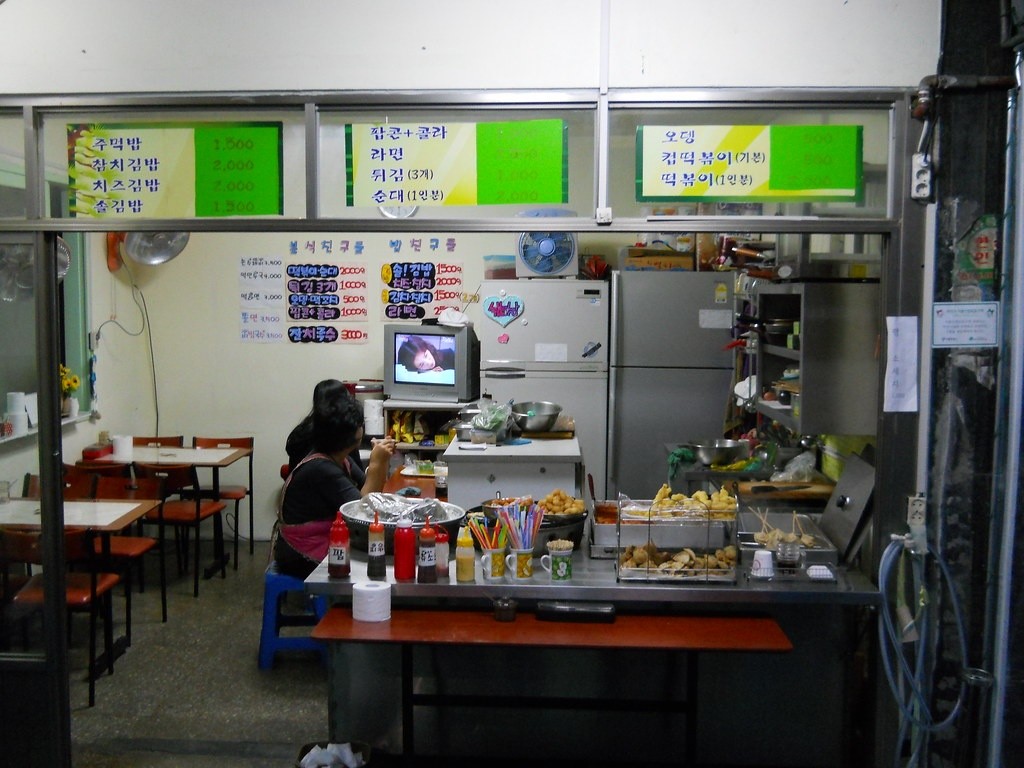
[751, 486, 812, 493]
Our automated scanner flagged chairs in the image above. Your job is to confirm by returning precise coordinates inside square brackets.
[0, 436, 255, 708]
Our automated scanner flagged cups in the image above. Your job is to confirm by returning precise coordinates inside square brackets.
[0, 481, 10, 505]
[540, 548, 572, 581]
[505, 545, 535, 581]
[481, 546, 507, 580]
[751, 550, 775, 577]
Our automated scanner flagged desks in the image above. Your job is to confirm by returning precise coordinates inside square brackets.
[0, 497, 162, 678]
[311, 603, 795, 768]
[99, 443, 251, 582]
[380, 465, 448, 504]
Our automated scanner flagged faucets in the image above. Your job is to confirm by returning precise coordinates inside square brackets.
[890, 532, 916, 560]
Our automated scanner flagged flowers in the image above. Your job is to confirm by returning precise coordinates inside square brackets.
[60, 365, 81, 397]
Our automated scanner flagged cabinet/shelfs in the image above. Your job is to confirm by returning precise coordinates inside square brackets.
[736, 276, 883, 434]
[383, 399, 475, 449]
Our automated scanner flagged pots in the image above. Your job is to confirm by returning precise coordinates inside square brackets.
[480, 498, 586, 527]
[734, 312, 796, 347]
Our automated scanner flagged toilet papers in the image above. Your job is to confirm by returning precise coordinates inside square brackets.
[3, 413, 28, 434]
[113, 436, 133, 457]
[7, 392, 25, 413]
[352, 580, 392, 622]
[363, 399, 385, 435]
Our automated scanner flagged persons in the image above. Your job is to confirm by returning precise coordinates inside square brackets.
[271, 396, 394, 580]
[285, 379, 365, 490]
[398, 335, 455, 373]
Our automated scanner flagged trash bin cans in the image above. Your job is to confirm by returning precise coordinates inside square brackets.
[295, 739, 371, 768]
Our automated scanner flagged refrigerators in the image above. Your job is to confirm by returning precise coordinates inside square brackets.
[607, 269, 734, 500]
[480, 279, 608, 504]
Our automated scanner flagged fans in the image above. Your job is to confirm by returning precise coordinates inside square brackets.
[108, 231, 189, 270]
[57, 237, 72, 286]
[515, 208, 579, 279]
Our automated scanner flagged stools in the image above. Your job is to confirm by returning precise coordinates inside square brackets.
[258, 561, 333, 675]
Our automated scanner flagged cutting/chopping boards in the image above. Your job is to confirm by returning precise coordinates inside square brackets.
[738, 481, 835, 498]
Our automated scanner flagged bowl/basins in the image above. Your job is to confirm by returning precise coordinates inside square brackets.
[339, 499, 466, 546]
[460, 517, 588, 557]
[806, 565, 833, 579]
[688, 439, 742, 465]
[512, 402, 563, 432]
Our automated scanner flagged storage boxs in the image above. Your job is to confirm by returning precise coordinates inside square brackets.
[626, 245, 694, 273]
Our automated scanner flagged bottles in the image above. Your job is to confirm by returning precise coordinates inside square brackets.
[367, 512, 386, 581]
[394, 509, 416, 583]
[435, 524, 450, 577]
[455, 526, 475, 582]
[328, 511, 351, 578]
[418, 516, 438, 582]
[435, 464, 448, 503]
[775, 545, 807, 580]
[497, 398, 514, 416]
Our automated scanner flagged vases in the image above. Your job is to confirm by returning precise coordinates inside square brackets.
[61, 395, 80, 417]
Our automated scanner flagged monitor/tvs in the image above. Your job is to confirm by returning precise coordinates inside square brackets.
[383, 324, 481, 403]
